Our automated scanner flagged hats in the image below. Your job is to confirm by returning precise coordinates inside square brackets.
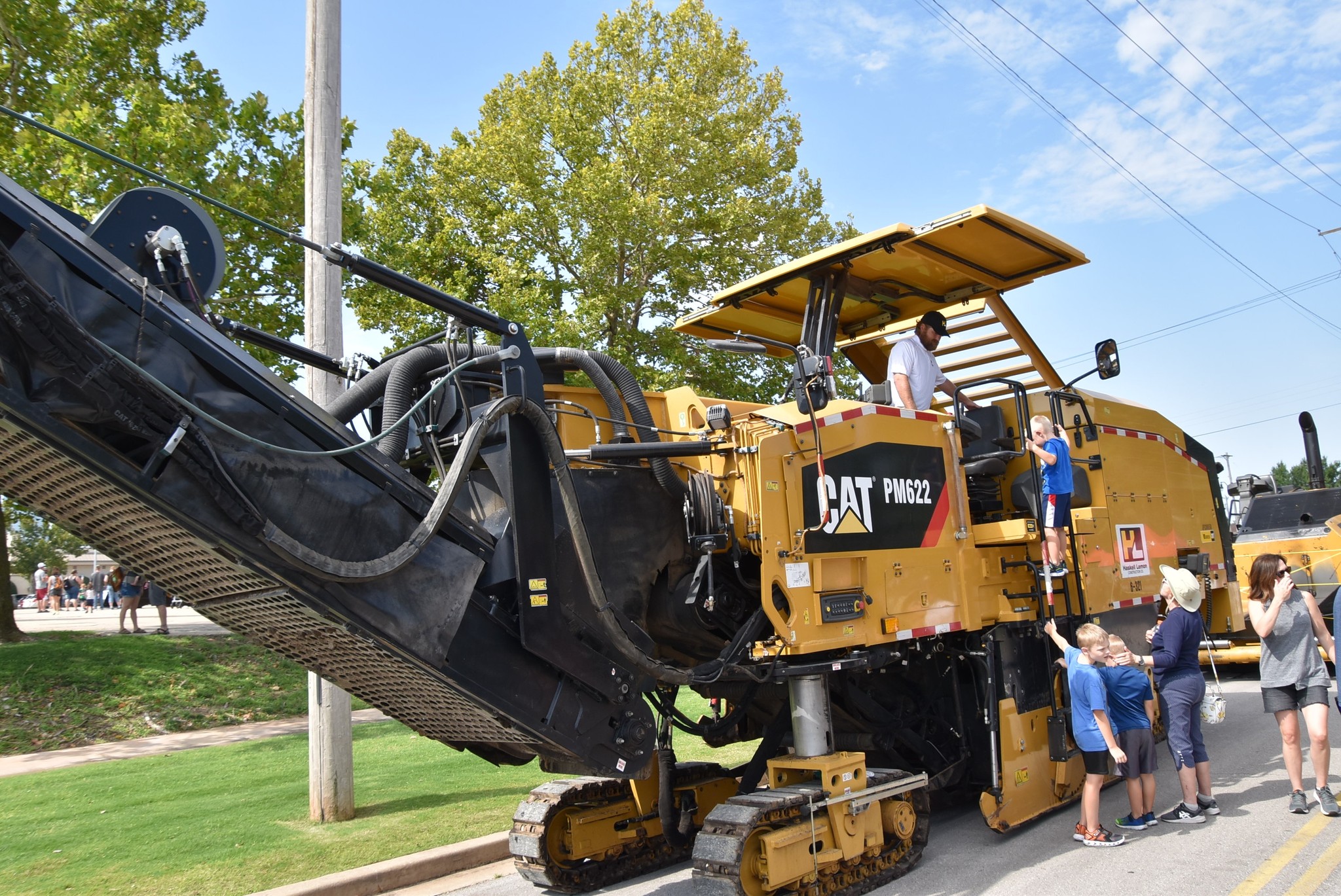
[37, 562, 46, 568]
[1159, 564, 1202, 612]
[921, 311, 951, 337]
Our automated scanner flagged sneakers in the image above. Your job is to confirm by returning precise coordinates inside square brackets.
[1287, 789, 1309, 813]
[1059, 560, 1069, 573]
[1115, 812, 1148, 830]
[1073, 821, 1087, 840]
[1313, 784, 1341, 815]
[1037, 561, 1064, 577]
[1197, 797, 1221, 815]
[1160, 802, 1206, 824]
[1141, 811, 1158, 826]
[1083, 824, 1125, 847]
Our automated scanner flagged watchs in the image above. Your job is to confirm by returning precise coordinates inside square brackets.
[1138, 655, 1145, 666]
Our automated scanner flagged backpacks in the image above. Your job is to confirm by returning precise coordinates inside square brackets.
[53, 575, 64, 590]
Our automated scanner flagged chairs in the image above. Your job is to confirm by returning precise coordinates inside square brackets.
[959, 406, 1012, 478]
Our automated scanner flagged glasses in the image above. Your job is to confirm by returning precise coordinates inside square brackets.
[1162, 578, 1169, 585]
[1275, 566, 1292, 578]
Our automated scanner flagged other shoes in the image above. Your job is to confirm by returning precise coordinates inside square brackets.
[38, 604, 123, 615]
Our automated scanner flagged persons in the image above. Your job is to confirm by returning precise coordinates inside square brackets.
[1025, 414, 1074, 577]
[118, 564, 170, 635]
[1247, 554, 1341, 816]
[886, 310, 983, 412]
[34, 561, 123, 612]
[1045, 564, 1220, 846]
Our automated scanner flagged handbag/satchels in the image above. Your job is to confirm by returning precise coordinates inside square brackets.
[64, 579, 70, 591]
[109, 567, 124, 592]
[1200, 684, 1227, 724]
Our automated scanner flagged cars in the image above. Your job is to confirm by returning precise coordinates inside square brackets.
[11, 591, 68, 609]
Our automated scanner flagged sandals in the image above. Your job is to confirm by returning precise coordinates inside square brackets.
[151, 628, 170, 635]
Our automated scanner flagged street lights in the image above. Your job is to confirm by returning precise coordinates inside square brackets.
[81, 546, 98, 572]
[1219, 480, 1239, 524]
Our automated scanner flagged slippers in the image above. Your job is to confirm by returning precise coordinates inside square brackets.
[119, 628, 131, 633]
[133, 628, 146, 633]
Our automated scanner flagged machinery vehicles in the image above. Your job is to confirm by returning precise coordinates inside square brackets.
[1197, 411, 1341, 666]
[1, 94, 1247, 896]
